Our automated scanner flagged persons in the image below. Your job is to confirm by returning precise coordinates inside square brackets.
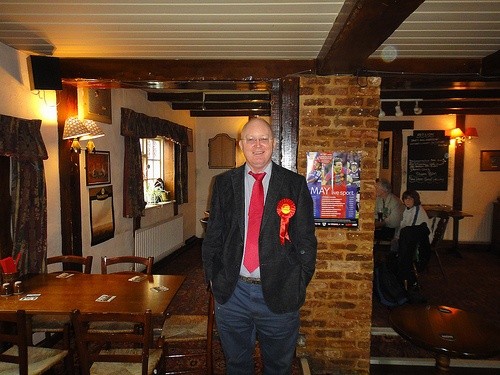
[383, 188, 435, 302]
[374, 177, 405, 229]
[201, 117, 317, 375]
[306, 156, 360, 186]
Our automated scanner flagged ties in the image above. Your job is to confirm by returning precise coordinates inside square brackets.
[243, 171, 267, 274]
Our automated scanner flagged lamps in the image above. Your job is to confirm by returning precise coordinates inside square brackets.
[450, 127, 478, 147]
[63, 116, 104, 154]
[414, 101, 422, 115]
[395, 101, 403, 116]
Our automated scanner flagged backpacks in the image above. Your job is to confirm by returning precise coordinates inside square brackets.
[376, 260, 409, 307]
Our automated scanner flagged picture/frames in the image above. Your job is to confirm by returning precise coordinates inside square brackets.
[84, 151, 111, 186]
[480, 150, 500, 171]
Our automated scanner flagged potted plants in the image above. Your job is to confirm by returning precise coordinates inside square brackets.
[153, 188, 171, 201]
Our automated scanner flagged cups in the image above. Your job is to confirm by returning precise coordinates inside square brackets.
[377, 208, 384, 222]
[1, 270, 20, 291]
[0, 283, 12, 296]
[13, 281, 24, 295]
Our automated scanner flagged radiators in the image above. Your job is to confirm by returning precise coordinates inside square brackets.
[135, 215, 183, 271]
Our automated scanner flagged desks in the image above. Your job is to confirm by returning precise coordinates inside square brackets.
[374, 226, 395, 241]
[0, 273, 187, 375]
[421, 204, 474, 259]
[389, 303, 500, 375]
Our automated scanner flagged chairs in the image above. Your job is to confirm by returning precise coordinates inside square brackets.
[384, 217, 449, 302]
[161, 292, 214, 375]
[72, 308, 162, 375]
[31, 255, 93, 351]
[0, 309, 69, 375]
[87, 255, 154, 333]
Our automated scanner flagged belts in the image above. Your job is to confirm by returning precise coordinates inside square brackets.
[239, 275, 261, 284]
